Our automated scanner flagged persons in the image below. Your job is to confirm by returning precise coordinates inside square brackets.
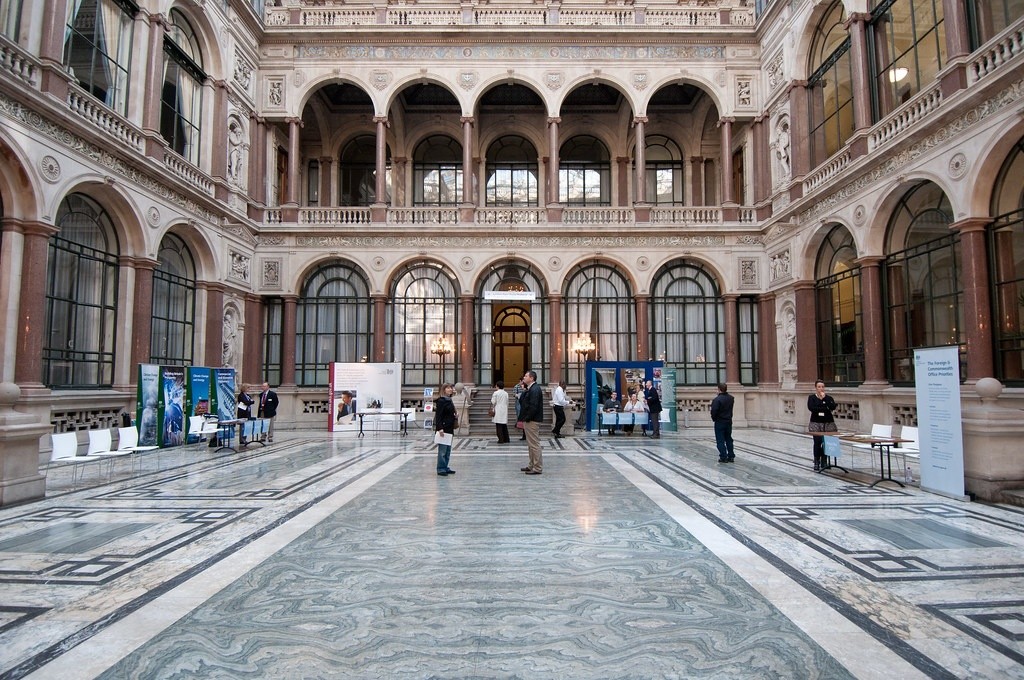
[435, 383, 459, 476]
[233, 253, 249, 280]
[603, 391, 622, 434]
[552, 380, 571, 438]
[807, 380, 837, 471]
[769, 251, 790, 280]
[230, 127, 242, 179]
[223, 314, 234, 364]
[258, 382, 279, 442]
[490, 382, 510, 444]
[337, 391, 356, 421]
[237, 383, 255, 443]
[741, 82, 750, 105]
[518, 371, 543, 474]
[776, 126, 789, 174]
[781, 314, 796, 366]
[270, 82, 281, 104]
[710, 384, 735, 463]
[623, 393, 645, 436]
[645, 380, 662, 439]
[514, 376, 528, 440]
[165, 386, 184, 445]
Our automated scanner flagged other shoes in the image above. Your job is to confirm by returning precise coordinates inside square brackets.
[821, 464, 831, 469]
[728, 457, 734, 462]
[814, 464, 819, 470]
[650, 433, 660, 439]
[525, 470, 542, 474]
[719, 458, 728, 463]
[519, 438, 526, 440]
[497, 439, 510, 443]
[446, 470, 455, 474]
[438, 472, 448, 476]
[551, 431, 565, 438]
[521, 467, 530, 471]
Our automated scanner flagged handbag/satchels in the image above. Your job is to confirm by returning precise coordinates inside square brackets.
[453, 415, 459, 429]
[209, 436, 223, 446]
[517, 421, 524, 428]
[488, 403, 495, 417]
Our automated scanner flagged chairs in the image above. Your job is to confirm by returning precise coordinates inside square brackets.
[184, 416, 217, 452]
[882, 426, 919, 483]
[202, 417, 228, 449]
[80, 429, 135, 483]
[111, 425, 161, 475]
[45, 432, 102, 490]
[851, 424, 892, 472]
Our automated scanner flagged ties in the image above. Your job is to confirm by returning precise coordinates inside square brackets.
[262, 394, 266, 409]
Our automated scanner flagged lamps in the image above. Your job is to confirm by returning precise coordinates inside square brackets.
[890, 67, 910, 83]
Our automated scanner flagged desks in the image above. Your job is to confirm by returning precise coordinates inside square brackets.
[807, 431, 915, 489]
[354, 412, 412, 437]
[597, 411, 650, 437]
[208, 415, 270, 454]
